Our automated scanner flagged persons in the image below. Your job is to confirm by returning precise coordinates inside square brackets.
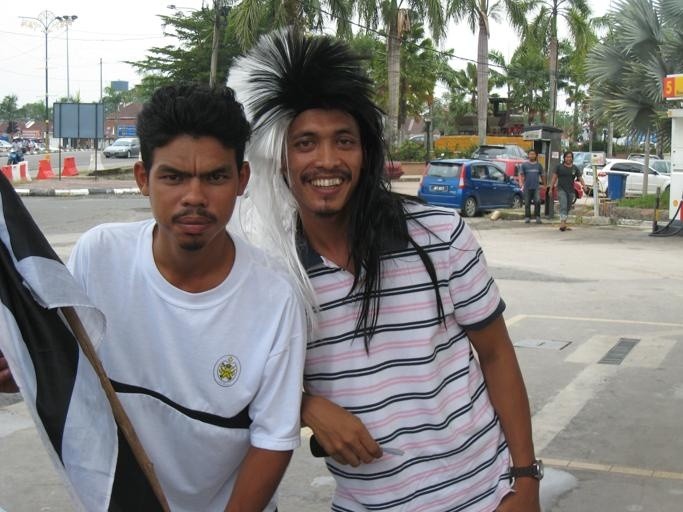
[548, 151, 588, 230]
[221, 25, 546, 509]
[518, 149, 549, 224]
[4, 137, 23, 162]
[0, 80, 309, 511]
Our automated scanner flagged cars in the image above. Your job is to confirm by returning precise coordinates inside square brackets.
[384, 159, 404, 183]
[404, 133, 441, 144]
[471, 143, 671, 205]
[418, 158, 524, 219]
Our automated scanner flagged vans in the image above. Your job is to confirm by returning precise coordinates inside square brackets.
[102, 138, 139, 158]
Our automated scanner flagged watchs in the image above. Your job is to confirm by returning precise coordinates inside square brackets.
[507, 459, 545, 481]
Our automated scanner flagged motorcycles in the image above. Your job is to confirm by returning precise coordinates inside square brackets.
[5, 150, 24, 165]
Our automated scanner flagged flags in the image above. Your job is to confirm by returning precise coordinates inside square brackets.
[0, 165, 121, 512]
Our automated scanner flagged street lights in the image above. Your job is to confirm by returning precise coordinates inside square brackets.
[422, 111, 433, 167]
[18, 11, 78, 171]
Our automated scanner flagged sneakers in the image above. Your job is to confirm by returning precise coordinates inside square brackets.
[525, 217, 530, 223]
[536, 217, 541, 224]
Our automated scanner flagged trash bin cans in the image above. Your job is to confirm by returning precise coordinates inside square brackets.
[606, 172, 629, 199]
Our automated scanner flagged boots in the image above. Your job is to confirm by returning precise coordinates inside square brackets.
[560, 220, 571, 231]
